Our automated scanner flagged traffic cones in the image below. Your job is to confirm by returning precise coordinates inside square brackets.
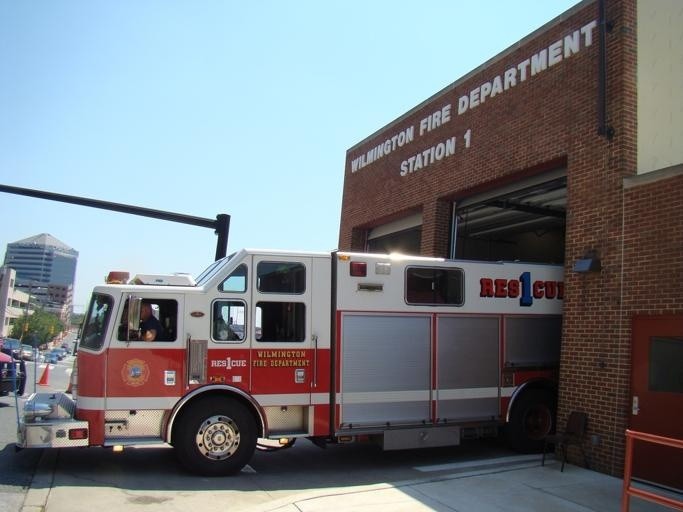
[36, 363, 51, 386]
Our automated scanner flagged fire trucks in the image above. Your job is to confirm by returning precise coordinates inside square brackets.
[14, 249, 564, 474]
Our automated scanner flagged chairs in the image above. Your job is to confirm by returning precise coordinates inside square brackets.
[540, 411, 589, 472]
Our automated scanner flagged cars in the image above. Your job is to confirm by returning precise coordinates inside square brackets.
[1, 350, 27, 396]
[3, 337, 72, 364]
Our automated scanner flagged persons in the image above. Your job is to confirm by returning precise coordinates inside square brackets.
[118, 301, 164, 341]
